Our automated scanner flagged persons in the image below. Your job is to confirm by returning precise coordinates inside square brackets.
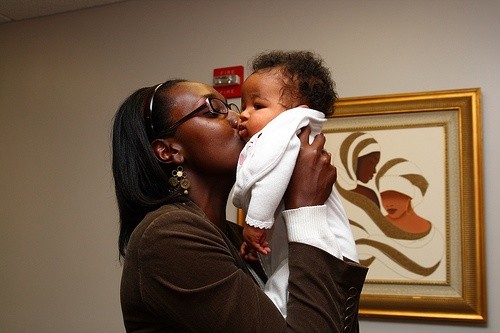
[231, 49, 361, 320]
[111, 78, 369, 333]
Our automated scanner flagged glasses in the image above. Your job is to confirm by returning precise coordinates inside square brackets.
[158, 97, 242, 139]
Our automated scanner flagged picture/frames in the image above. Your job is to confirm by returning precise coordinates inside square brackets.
[239, 84, 490, 324]
